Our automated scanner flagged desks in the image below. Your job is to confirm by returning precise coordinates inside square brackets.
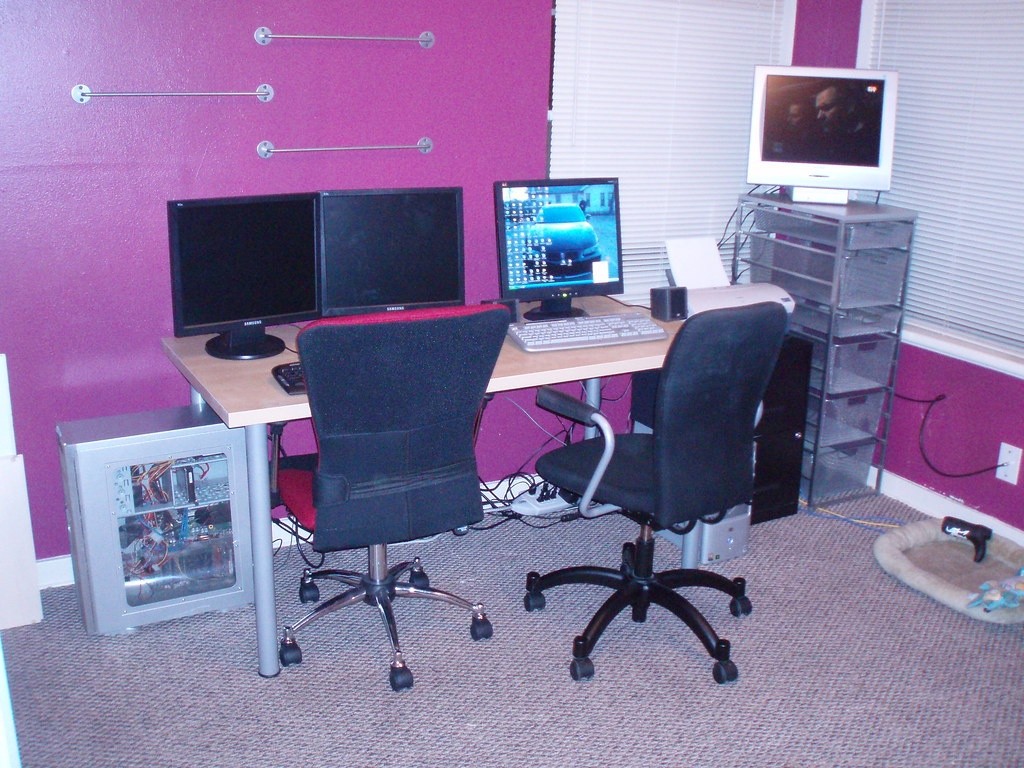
[158, 295, 701, 677]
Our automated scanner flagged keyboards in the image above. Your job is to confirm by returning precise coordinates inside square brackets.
[506, 311, 669, 352]
[271, 362, 306, 396]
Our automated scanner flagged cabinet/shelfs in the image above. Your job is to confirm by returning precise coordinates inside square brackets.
[731, 195, 921, 510]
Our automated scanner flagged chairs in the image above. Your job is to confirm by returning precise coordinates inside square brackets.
[267, 305, 515, 691]
[523, 301, 788, 686]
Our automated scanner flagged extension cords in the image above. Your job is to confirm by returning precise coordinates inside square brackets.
[510, 485, 581, 515]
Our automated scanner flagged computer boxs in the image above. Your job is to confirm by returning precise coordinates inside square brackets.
[57, 403, 255, 633]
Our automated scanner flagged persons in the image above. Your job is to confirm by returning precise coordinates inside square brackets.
[812, 82, 878, 167]
[780, 98, 824, 163]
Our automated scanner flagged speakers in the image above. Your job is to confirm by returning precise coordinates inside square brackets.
[480, 299, 517, 323]
[650, 286, 688, 321]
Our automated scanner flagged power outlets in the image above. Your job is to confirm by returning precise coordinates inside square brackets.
[995, 442, 1023, 485]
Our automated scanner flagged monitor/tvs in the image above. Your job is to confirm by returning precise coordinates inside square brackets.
[493, 177, 624, 320]
[165, 191, 321, 360]
[747, 65, 898, 206]
[318, 186, 465, 317]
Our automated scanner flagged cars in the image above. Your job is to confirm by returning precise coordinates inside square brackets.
[523, 203, 601, 275]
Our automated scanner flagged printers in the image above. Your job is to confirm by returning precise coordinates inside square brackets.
[664, 269, 794, 319]
